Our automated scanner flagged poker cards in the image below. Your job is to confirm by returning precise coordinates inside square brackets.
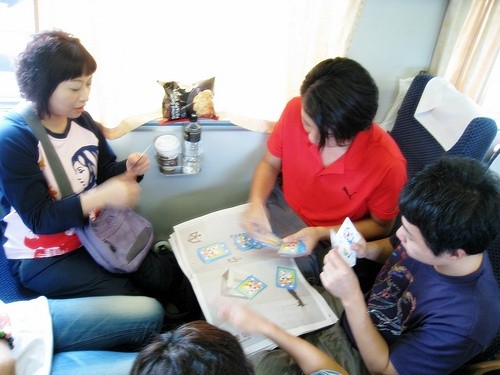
[329, 217, 361, 268]
[130, 143, 151, 169]
[235, 275, 267, 300]
[197, 242, 231, 264]
[278, 239, 307, 256]
[275, 266, 297, 291]
[231, 229, 277, 251]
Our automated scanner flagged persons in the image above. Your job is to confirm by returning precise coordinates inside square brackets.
[250, 157, 500, 375]
[130, 301, 350, 375]
[0, 30, 207, 332]
[241, 57, 407, 258]
[0, 296, 165, 375]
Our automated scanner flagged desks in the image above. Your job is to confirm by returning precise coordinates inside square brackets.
[104, 116, 273, 245]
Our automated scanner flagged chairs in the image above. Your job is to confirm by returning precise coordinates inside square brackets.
[389, 70, 498, 183]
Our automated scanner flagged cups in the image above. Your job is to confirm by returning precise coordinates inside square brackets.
[155, 135, 180, 175]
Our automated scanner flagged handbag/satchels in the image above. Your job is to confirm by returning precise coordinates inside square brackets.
[19, 102, 154, 274]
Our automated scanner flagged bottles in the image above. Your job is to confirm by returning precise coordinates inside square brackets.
[183, 114, 200, 174]
